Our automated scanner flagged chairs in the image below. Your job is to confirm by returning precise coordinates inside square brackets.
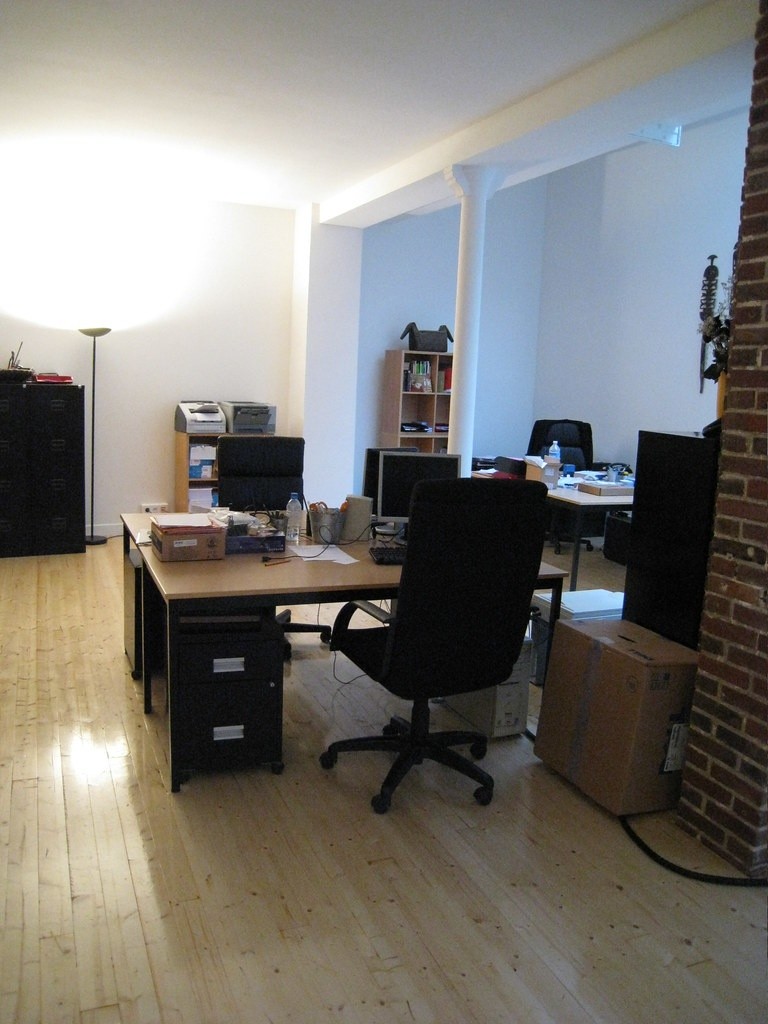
[526, 421, 599, 556]
[208, 433, 329, 657]
[319, 479, 556, 808]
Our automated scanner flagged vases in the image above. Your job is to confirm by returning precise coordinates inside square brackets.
[716, 370, 726, 416]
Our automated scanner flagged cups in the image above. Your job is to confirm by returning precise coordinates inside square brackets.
[563, 464, 575, 477]
[271, 516, 285, 531]
[607, 470, 618, 481]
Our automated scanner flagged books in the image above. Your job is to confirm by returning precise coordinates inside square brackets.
[437, 368, 451, 393]
[410, 360, 431, 375]
[404, 369, 411, 392]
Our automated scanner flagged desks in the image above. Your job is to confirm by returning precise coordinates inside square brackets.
[137, 548, 567, 797]
[540, 485, 636, 591]
[117, 503, 349, 684]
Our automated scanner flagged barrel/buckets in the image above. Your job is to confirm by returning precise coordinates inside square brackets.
[307, 507, 345, 544]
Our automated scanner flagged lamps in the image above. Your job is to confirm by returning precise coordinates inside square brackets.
[78, 325, 113, 544]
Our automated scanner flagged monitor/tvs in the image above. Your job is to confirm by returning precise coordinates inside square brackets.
[362, 448, 419, 514]
[378, 450, 461, 523]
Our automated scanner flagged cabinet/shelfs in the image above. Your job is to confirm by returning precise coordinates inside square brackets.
[380, 350, 454, 451]
[0, 384, 87, 560]
[174, 429, 279, 512]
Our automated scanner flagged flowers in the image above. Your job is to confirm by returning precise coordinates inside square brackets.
[698, 275, 736, 382]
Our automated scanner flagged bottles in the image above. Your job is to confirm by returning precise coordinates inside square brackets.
[287, 493, 301, 542]
[549, 441, 561, 461]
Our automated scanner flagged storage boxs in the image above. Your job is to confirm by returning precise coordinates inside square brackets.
[149, 522, 227, 562]
[531, 588, 699, 815]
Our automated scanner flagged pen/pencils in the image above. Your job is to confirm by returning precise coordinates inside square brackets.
[266, 508, 280, 519]
[265, 559, 292, 566]
[620, 464, 631, 474]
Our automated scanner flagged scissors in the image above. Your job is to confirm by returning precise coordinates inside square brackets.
[315, 501, 328, 511]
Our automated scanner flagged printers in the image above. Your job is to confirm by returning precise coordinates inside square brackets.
[174, 400, 226, 434]
[217, 401, 277, 435]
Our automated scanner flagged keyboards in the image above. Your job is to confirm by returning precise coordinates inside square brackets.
[369, 547, 407, 564]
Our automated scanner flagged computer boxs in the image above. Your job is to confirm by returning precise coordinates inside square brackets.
[446, 636, 533, 740]
